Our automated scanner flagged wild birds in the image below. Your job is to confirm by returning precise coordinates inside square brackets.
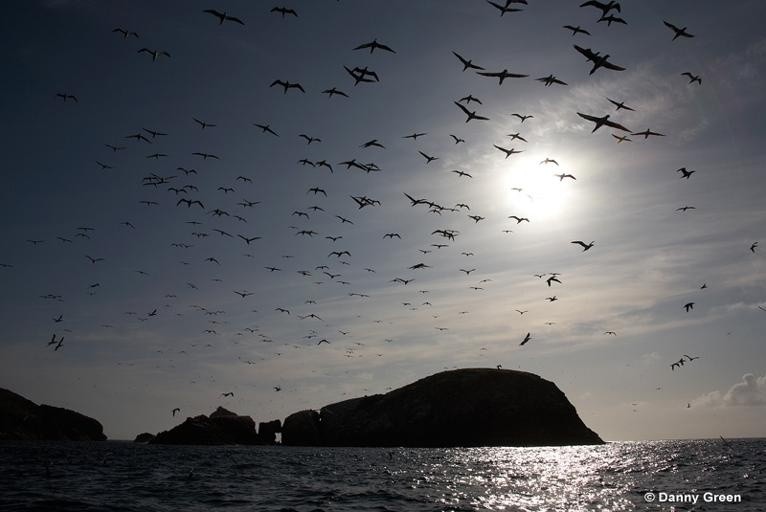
[720, 434, 735, 450]
[749, 240, 759, 255]
[171, 406, 180, 418]
[0, 0, 710, 400]
